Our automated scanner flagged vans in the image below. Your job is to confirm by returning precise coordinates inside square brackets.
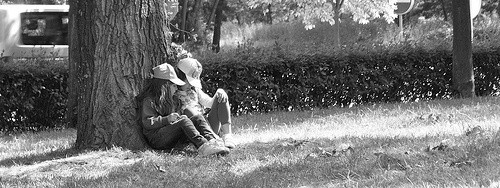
[0, 5, 70, 68]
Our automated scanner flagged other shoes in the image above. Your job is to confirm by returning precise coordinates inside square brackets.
[221, 134, 235, 148]
[197, 142, 225, 157]
[209, 138, 230, 154]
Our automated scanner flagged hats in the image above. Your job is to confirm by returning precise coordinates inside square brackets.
[150, 63, 186, 86]
[176, 58, 203, 89]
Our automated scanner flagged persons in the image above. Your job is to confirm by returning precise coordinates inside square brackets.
[171, 55, 236, 148]
[134, 60, 231, 156]
